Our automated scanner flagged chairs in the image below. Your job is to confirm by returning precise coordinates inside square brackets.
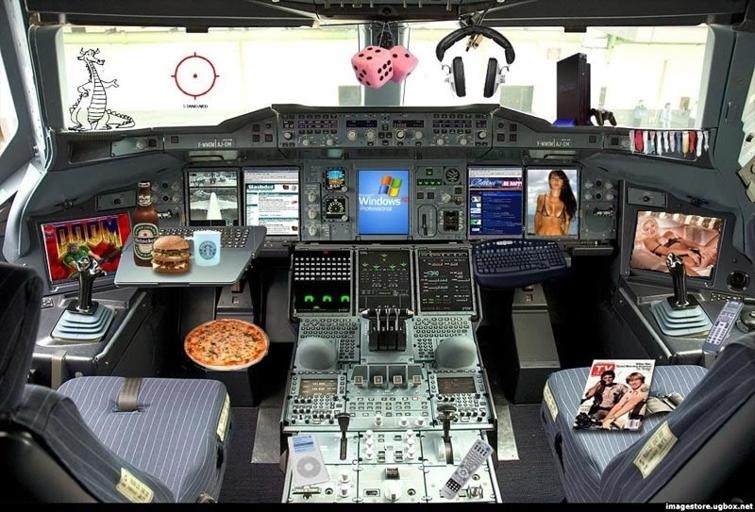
[540, 331, 755, 503]
[0, 261, 232, 503]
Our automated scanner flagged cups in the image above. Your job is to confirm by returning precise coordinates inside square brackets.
[194, 230, 221, 267]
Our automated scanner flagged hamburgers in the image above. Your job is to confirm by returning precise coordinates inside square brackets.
[151, 234, 190, 273]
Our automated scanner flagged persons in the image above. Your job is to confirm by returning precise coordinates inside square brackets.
[532, 170, 579, 236]
[657, 101, 675, 128]
[584, 369, 630, 425]
[629, 98, 647, 127]
[640, 215, 723, 277]
[598, 371, 650, 429]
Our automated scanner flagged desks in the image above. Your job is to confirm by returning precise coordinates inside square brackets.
[113, 226, 267, 288]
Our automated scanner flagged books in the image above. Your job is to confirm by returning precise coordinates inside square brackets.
[572, 357, 657, 433]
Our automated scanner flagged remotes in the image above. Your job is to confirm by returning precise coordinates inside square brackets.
[441, 439, 495, 500]
[701, 301, 745, 355]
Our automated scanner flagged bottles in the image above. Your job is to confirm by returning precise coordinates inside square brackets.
[132, 181, 158, 267]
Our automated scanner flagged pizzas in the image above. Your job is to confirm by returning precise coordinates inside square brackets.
[182, 318, 269, 370]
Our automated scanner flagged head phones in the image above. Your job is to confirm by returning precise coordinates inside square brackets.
[436, 25, 516, 98]
